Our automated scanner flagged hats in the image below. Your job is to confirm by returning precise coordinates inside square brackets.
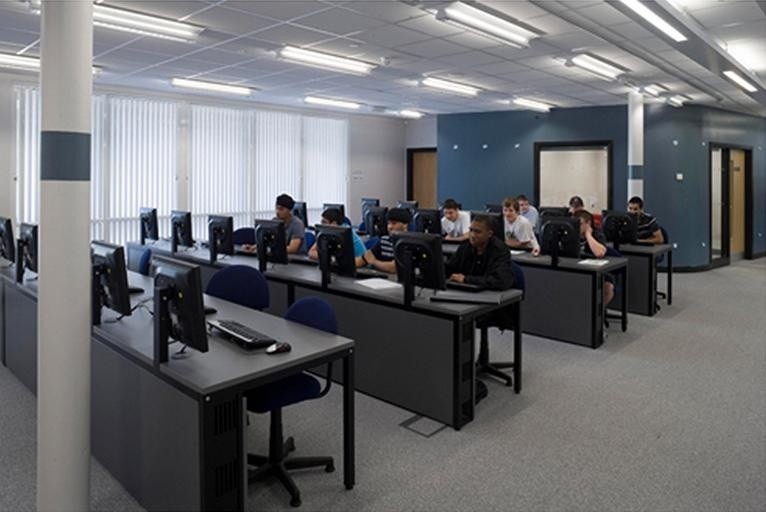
[276, 194, 295, 210]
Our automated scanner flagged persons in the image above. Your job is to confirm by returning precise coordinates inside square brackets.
[531, 209, 616, 330]
[240, 193, 306, 255]
[307, 207, 368, 269]
[516, 194, 541, 244]
[355, 207, 413, 274]
[448, 213, 514, 332]
[626, 195, 664, 264]
[500, 196, 539, 253]
[440, 198, 471, 242]
[567, 195, 584, 215]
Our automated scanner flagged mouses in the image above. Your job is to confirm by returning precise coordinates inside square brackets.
[265, 342, 290, 355]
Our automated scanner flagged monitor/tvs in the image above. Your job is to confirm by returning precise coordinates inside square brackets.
[361, 198, 380, 222]
[323, 204, 344, 217]
[207, 214, 234, 261]
[438, 202, 463, 216]
[486, 204, 503, 213]
[362, 205, 388, 237]
[15, 223, 37, 283]
[389, 230, 447, 306]
[602, 210, 637, 250]
[255, 219, 289, 272]
[151, 253, 209, 363]
[315, 224, 357, 289]
[397, 200, 419, 217]
[470, 210, 505, 242]
[539, 215, 582, 266]
[413, 208, 442, 233]
[171, 211, 193, 252]
[539, 207, 571, 216]
[0, 216, 15, 263]
[291, 202, 308, 227]
[90, 239, 132, 325]
[140, 208, 159, 244]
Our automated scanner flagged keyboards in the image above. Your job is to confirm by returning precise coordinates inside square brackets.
[207, 319, 277, 350]
[235, 251, 257, 257]
[509, 246, 533, 252]
[445, 278, 482, 293]
[580, 250, 595, 259]
[288, 259, 318, 265]
[204, 309, 216, 315]
[631, 242, 655, 246]
[357, 266, 388, 277]
[130, 288, 145, 293]
